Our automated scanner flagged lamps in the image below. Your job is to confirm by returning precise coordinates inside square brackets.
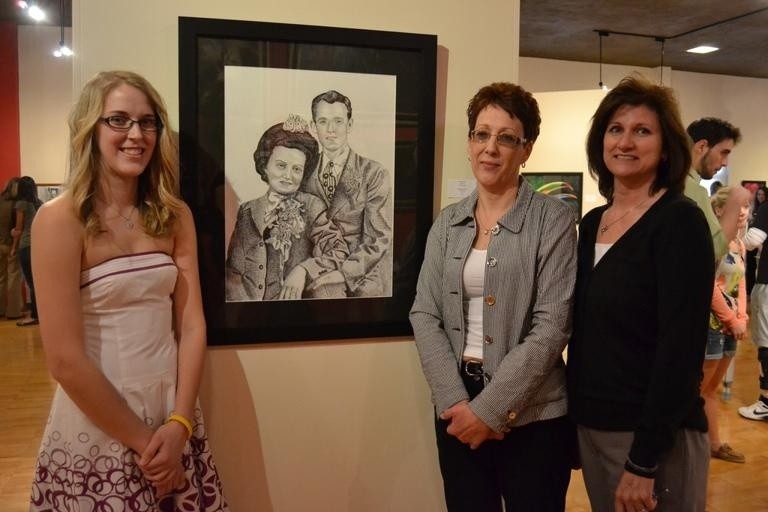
[597, 33, 609, 92]
[51, 1, 74, 60]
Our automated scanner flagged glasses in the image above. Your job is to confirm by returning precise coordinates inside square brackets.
[97, 115, 162, 132]
[469, 126, 529, 148]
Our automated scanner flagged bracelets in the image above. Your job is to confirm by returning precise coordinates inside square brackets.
[163, 414, 194, 441]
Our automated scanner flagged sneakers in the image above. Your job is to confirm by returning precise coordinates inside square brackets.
[738, 399, 768, 421]
[710, 441, 747, 464]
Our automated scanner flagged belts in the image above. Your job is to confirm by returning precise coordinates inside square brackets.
[459, 359, 484, 377]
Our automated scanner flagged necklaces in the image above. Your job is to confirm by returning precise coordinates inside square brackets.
[600, 192, 652, 234]
[474, 206, 507, 236]
[91, 191, 143, 230]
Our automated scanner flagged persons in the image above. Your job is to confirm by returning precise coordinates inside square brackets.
[28, 69, 229, 512]
[696, 183, 768, 468]
[566, 77, 718, 512]
[222, 116, 351, 302]
[0, 176, 25, 321]
[304, 90, 394, 299]
[664, 117, 752, 275]
[8, 176, 51, 325]
[408, 82, 581, 510]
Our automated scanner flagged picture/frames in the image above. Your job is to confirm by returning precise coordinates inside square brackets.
[522, 171, 582, 224]
[36, 184, 64, 203]
[741, 180, 766, 192]
[177, 15, 437, 347]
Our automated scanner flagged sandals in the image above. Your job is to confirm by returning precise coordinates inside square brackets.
[6, 312, 39, 327]
